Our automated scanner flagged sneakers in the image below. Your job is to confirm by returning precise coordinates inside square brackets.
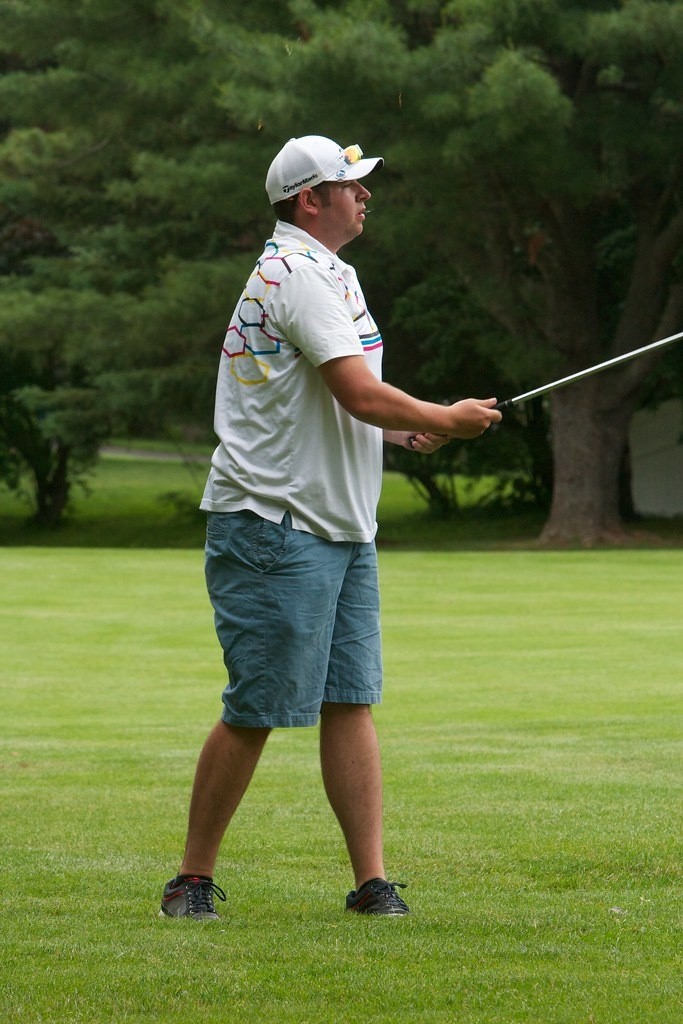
[346, 878, 410, 916]
[159, 879, 226, 920]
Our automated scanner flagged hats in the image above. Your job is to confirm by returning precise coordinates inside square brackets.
[266, 135, 384, 205]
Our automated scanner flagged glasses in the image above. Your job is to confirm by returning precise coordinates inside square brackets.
[342, 144, 363, 166]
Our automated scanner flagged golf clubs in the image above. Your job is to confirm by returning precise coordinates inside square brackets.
[406, 331, 683, 450]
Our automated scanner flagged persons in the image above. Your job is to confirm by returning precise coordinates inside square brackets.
[160, 134, 502, 920]
